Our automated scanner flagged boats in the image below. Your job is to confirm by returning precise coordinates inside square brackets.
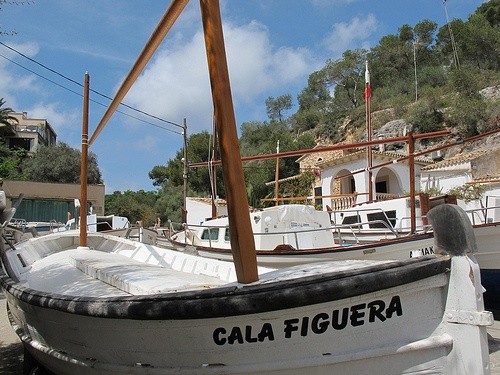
[0, 229, 455, 375]
[162, 191, 500, 305]
